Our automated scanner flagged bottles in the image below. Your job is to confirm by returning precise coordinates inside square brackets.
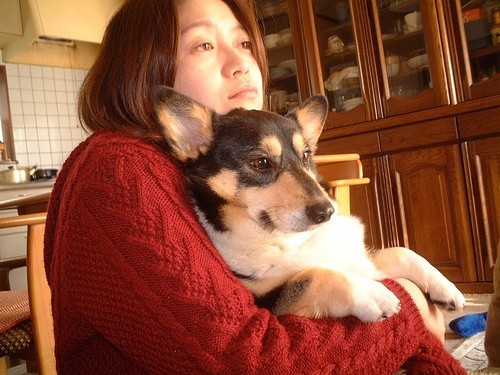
[490, 11, 500, 46]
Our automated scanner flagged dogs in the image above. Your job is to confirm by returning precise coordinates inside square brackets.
[149, 85, 466, 323]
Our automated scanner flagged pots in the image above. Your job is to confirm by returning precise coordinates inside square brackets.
[0, 165, 37, 185]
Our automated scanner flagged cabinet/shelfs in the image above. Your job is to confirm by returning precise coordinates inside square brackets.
[257, 1, 499, 295]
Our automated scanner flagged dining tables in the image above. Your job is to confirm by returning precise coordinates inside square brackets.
[0, 153, 373, 216]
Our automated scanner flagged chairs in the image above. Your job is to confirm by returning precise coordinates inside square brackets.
[0, 213, 56, 375]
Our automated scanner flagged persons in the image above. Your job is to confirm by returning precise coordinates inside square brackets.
[44, 1, 469, 375]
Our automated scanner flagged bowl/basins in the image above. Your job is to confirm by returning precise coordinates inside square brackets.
[32, 169, 58, 180]
[404, 12, 421, 26]
[345, 78, 359, 86]
[382, 47, 428, 75]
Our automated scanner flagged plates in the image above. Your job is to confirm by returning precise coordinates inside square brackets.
[270, 58, 295, 78]
[382, 34, 396, 40]
[263, 28, 292, 48]
[341, 97, 363, 112]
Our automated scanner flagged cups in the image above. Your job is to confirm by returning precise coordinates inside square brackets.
[269, 90, 287, 116]
[389, 85, 419, 97]
[392, 19, 403, 36]
[327, 35, 343, 53]
[335, 2, 348, 24]
